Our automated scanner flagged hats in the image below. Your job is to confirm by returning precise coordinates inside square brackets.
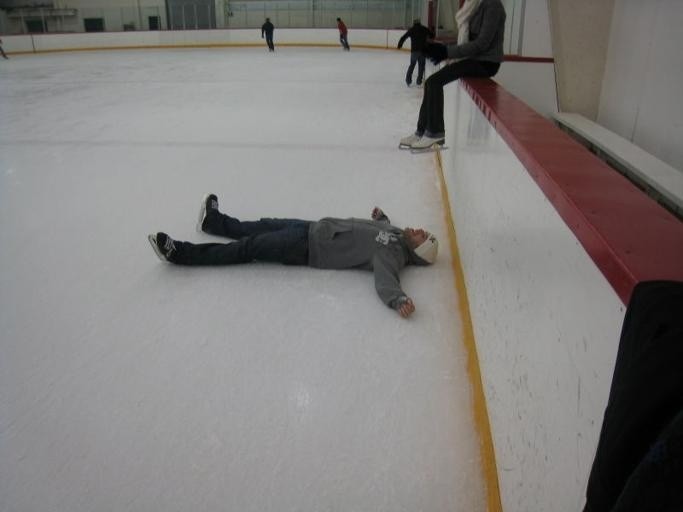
[414, 233, 438, 264]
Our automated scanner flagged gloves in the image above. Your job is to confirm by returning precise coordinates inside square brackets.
[423, 42, 448, 65]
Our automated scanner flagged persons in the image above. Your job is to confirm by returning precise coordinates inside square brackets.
[397, 19, 430, 88]
[148, 191, 437, 316]
[261, 18, 275, 52]
[336, 17, 349, 51]
[396, 1, 506, 153]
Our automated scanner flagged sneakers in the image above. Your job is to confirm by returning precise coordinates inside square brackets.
[202, 195, 220, 234]
[157, 232, 177, 261]
[400, 134, 421, 145]
[411, 135, 445, 148]
